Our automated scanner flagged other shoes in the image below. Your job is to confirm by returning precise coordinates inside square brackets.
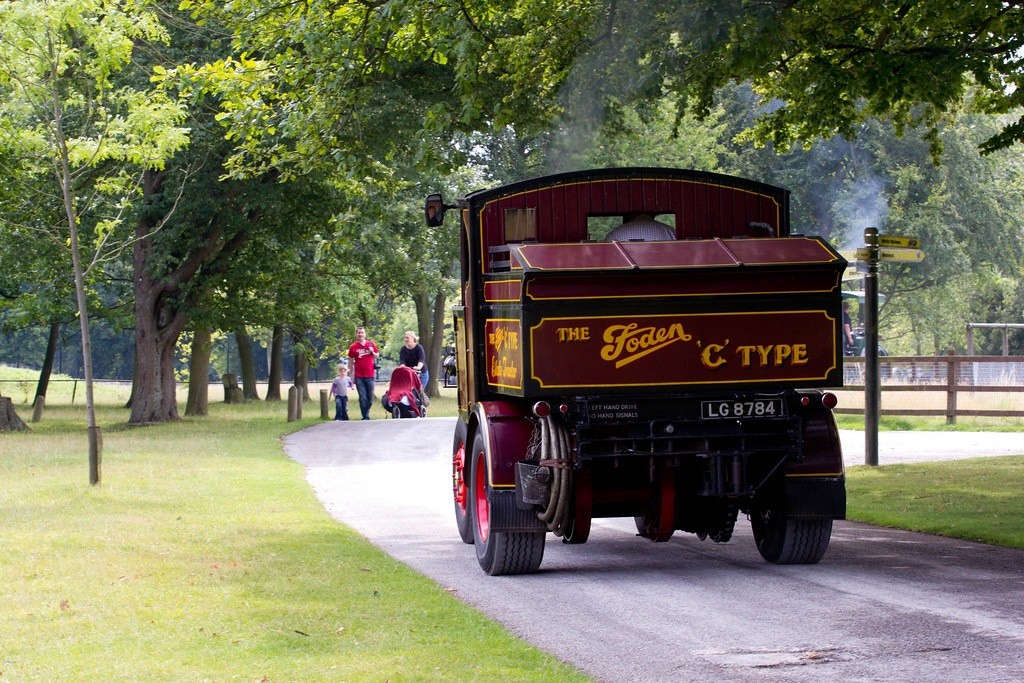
[361, 416, 369, 420]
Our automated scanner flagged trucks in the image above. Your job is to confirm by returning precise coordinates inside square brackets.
[426, 167, 849, 577]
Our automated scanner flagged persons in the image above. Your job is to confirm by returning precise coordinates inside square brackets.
[400, 331, 429, 390]
[843, 301, 854, 356]
[604, 213, 676, 242]
[332, 364, 354, 421]
[348, 327, 379, 420]
[860, 334, 892, 380]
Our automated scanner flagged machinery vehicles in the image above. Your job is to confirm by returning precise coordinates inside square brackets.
[840, 288, 892, 380]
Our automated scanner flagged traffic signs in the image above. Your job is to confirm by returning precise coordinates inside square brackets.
[835, 238, 927, 284]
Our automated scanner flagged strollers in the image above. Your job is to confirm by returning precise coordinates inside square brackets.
[381, 367, 430, 419]
